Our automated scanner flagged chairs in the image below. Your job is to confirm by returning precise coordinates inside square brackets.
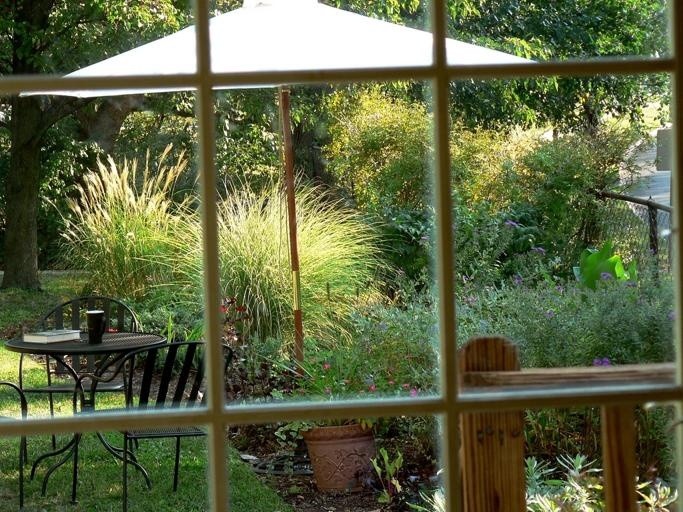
[18, 295, 144, 462]
[1, 381, 29, 511]
[70, 340, 235, 503]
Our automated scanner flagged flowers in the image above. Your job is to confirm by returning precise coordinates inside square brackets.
[258, 340, 384, 496]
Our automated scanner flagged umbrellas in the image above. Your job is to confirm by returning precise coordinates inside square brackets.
[14, 0, 543, 366]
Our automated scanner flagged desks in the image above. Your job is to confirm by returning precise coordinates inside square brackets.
[5, 330, 167, 498]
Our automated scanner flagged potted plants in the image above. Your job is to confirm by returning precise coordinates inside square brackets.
[296, 424, 378, 494]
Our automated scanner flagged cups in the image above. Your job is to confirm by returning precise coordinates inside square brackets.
[86, 310, 106, 344]
